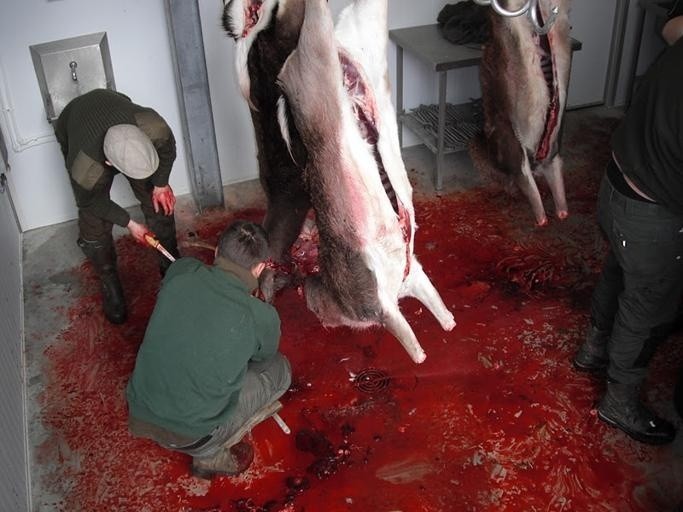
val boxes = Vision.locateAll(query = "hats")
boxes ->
[103,124,159,180]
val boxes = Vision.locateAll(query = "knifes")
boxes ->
[145,234,176,264]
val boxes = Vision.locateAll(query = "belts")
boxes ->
[165,426,219,451]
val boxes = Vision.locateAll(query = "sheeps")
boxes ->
[221,0,311,304]
[478,0,571,228]
[276,0,457,365]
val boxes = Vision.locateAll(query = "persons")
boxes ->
[125,222,293,478]
[571,15,683,444]
[53,88,183,325]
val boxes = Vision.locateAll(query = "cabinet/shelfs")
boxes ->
[388,25,583,193]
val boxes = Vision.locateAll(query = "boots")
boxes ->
[191,400,283,480]
[573,334,610,371]
[597,379,676,445]
[77,237,127,325]
[151,232,181,280]
